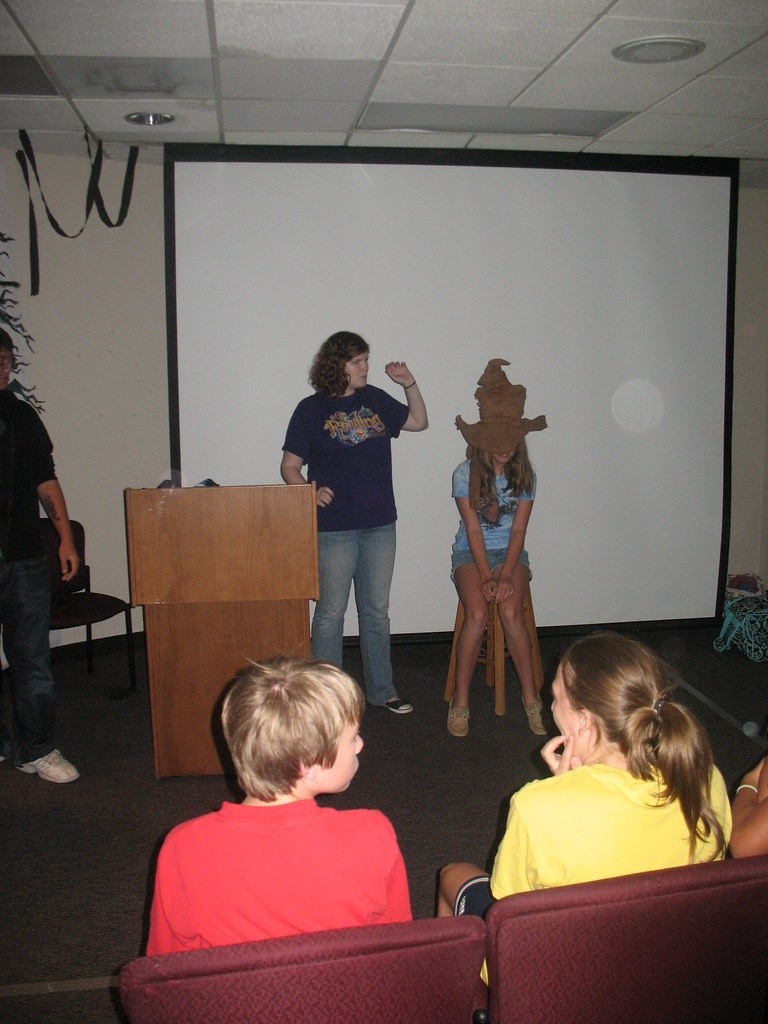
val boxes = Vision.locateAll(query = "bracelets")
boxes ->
[736,785,758,793]
[405,381,415,389]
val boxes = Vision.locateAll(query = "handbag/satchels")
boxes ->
[721,573,768,617]
[713,594,768,663]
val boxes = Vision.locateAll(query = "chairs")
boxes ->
[37,516,139,694]
[120,913,486,1024]
[486,854,768,1024]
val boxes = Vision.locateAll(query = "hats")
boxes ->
[453,359,548,455]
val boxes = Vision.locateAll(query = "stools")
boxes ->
[444,583,543,717]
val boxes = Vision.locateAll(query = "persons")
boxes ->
[447,412,553,736]
[435,633,733,985]
[144,657,413,953]
[281,330,429,713]
[0,329,79,783]
[729,756,768,859]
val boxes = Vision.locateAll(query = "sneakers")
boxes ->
[14,749,79,783]
[447,696,470,737]
[521,692,553,735]
[383,699,413,713]
